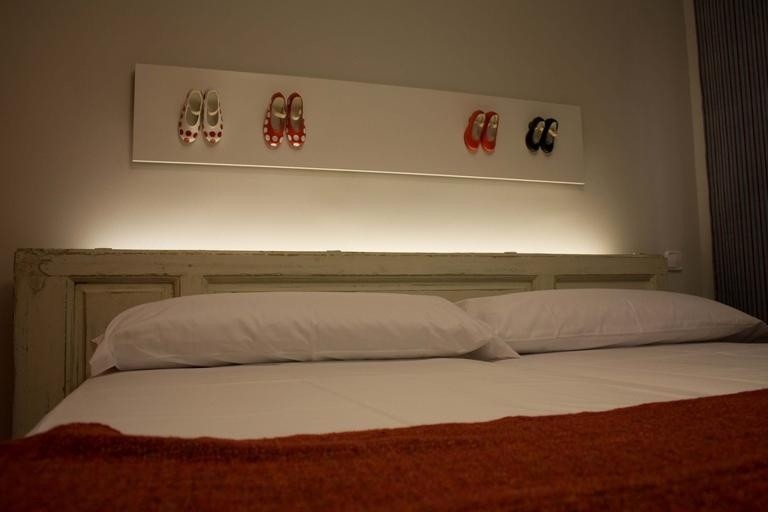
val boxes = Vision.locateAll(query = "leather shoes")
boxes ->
[263,92,287,150]
[541,119,558,153]
[178,88,204,143]
[464,110,486,151]
[203,88,223,144]
[481,112,499,152]
[286,92,306,148]
[525,117,546,152]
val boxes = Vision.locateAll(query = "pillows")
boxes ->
[451,288,768,359]
[86,289,523,380]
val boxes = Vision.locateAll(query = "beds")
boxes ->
[6,244,768,512]
